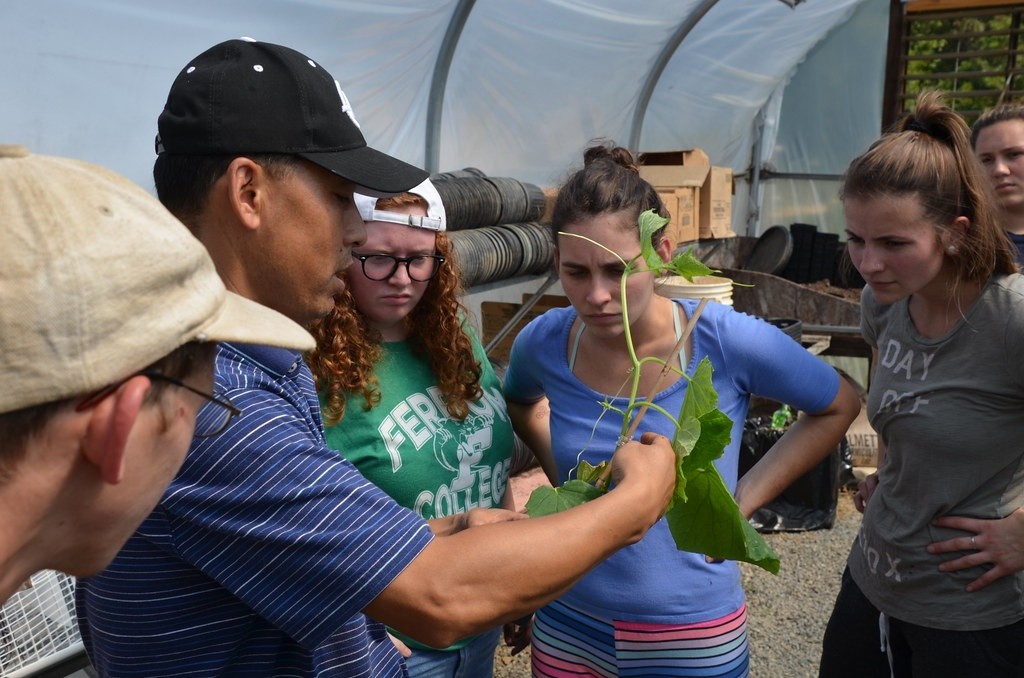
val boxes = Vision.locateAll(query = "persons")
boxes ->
[501,138,860,678]
[77,37,676,678]
[818,87,1024,678]
[0,144,316,609]
[303,176,532,678]
[970,105,1024,274]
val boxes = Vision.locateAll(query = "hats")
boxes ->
[154,37,431,197]
[352,175,447,232]
[0,144,316,415]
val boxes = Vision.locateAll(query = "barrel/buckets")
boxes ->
[653,275,734,308]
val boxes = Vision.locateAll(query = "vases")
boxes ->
[741,317,842,532]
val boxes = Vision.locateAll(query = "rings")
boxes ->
[972,537,977,552]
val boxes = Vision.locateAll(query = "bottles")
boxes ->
[771,403,793,429]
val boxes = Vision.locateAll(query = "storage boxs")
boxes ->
[634,146,737,247]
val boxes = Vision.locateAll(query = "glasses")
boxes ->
[350,250,444,283]
[77,371,241,438]
